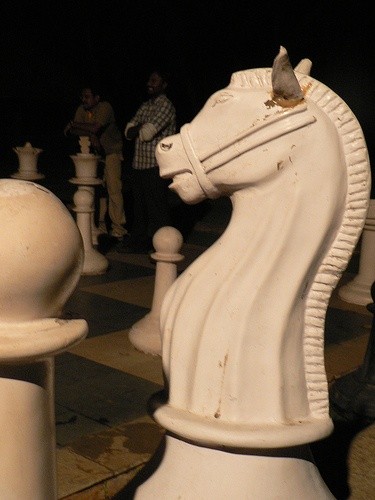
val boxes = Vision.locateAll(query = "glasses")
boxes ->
[80,95,93,99]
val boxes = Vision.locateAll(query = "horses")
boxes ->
[155,45,371,448]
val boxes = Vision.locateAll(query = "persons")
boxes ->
[64,84,129,247]
[116,69,178,253]
